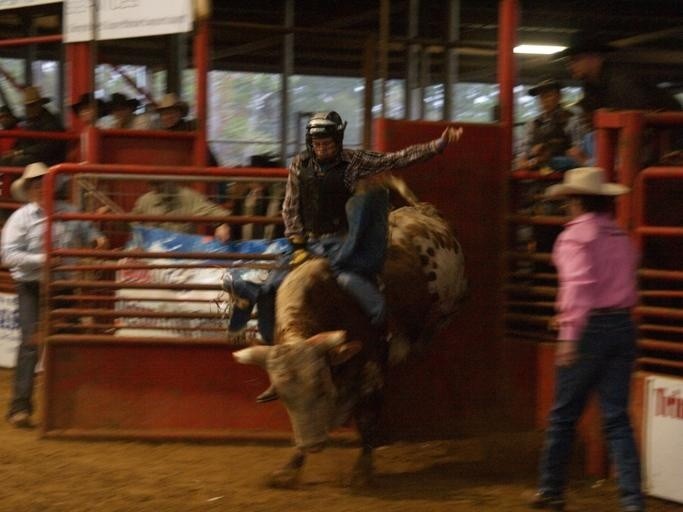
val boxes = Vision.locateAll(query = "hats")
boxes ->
[15,85,51,107]
[71,92,190,117]
[528,77,568,96]
[11,162,61,200]
[544,167,632,200]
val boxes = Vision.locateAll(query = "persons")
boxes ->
[518,74,583,330]
[528,166,644,512]
[230,154,286,340]
[0,82,198,166]
[0,162,111,427]
[135,175,234,245]
[557,32,682,161]
[261,109,465,397]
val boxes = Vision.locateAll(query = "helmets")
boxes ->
[306,110,347,137]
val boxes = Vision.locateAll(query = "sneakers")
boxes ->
[256,384,279,403]
[528,487,564,509]
[385,328,413,368]
[7,408,30,429]
[621,494,645,512]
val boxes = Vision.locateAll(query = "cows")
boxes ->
[229,174,468,497]
